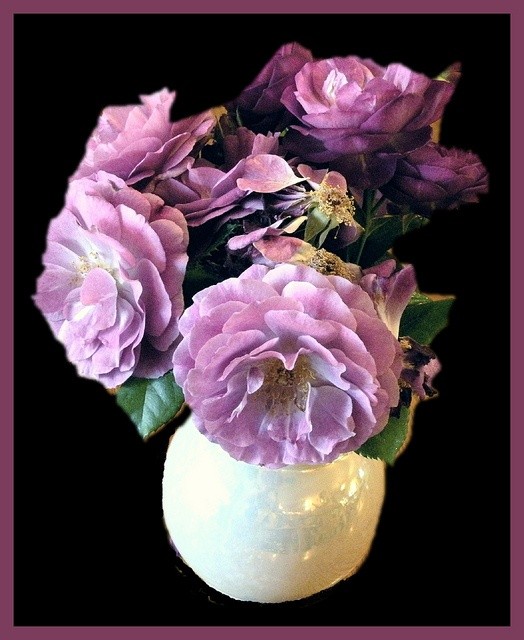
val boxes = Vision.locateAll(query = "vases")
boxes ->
[160,413,386,605]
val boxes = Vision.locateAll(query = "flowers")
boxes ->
[31,44,488,466]
